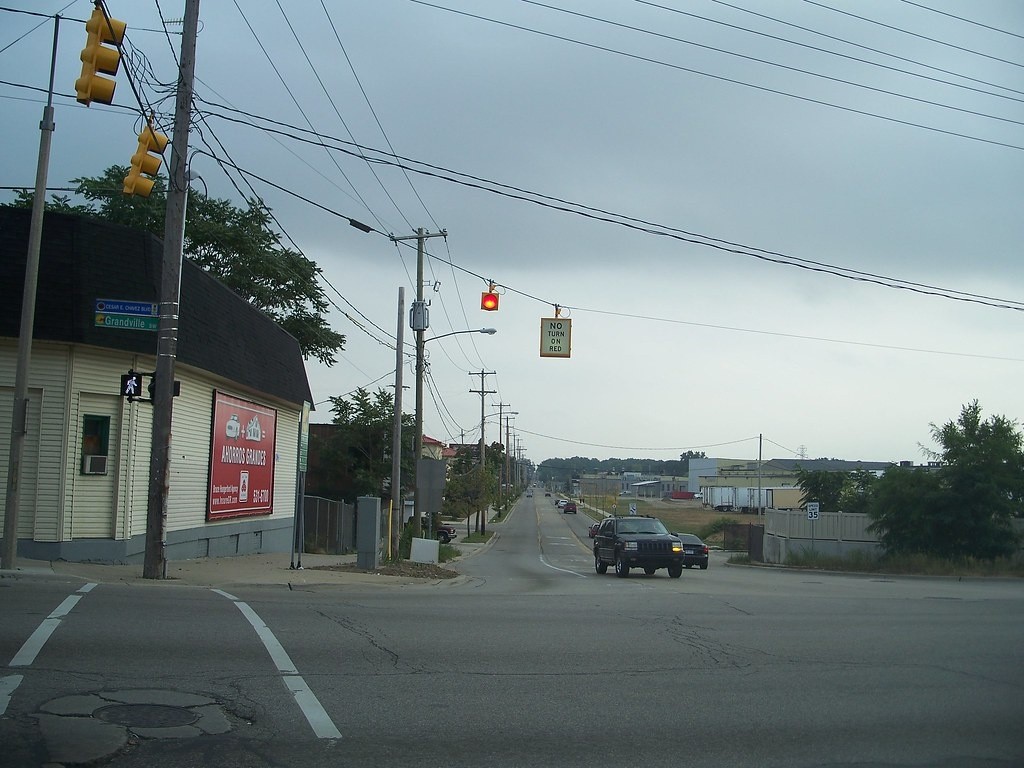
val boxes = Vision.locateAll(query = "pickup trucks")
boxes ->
[405,515,458,544]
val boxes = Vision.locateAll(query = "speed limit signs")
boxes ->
[807,503,820,520]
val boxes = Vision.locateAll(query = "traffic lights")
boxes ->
[483,291,497,311]
[121,376,143,396]
[74,6,125,108]
[121,117,168,197]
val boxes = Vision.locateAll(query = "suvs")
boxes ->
[564,502,577,514]
[594,515,685,578]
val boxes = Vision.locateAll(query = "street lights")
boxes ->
[413,326,497,533]
[481,411,519,534]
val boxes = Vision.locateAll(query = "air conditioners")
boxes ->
[83,454,108,475]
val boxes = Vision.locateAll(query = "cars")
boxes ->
[557,500,568,508]
[588,524,602,537]
[693,491,703,499]
[554,500,561,505]
[671,531,710,570]
[527,484,552,499]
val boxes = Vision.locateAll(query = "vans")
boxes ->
[618,491,631,496]
